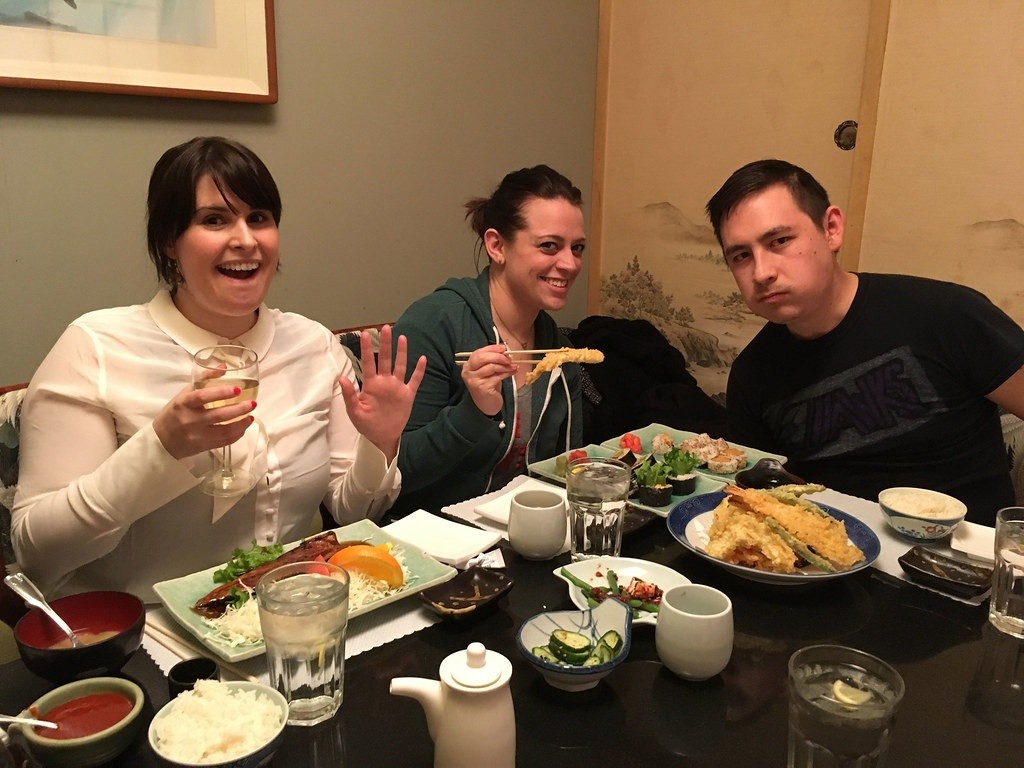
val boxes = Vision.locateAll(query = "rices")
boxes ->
[153,677,283,764]
[881,490,960,519]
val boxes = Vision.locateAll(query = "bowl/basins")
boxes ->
[147,680,290,768]
[878,487,968,545]
[515,596,633,693]
[13,591,148,684]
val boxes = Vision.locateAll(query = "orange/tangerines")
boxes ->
[326,545,403,589]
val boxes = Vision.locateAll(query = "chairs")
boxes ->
[0,322,398,665]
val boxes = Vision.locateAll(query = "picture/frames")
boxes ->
[0,0,279,106]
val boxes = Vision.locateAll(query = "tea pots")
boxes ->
[389,642,516,768]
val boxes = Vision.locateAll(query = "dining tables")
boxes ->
[0,480,1024,768]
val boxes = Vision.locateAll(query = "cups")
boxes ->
[988,506,1024,640]
[787,645,905,768]
[653,583,733,679]
[508,490,567,562]
[3,677,155,768]
[566,457,631,563]
[169,657,219,700]
[256,561,350,727]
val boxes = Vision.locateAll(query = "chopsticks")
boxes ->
[454,349,567,365]
[143,619,255,680]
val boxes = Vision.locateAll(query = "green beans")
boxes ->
[561,567,660,618]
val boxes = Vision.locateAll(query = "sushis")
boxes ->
[651,433,747,475]
[609,448,698,506]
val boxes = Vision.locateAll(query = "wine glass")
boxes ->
[195,345,255,498]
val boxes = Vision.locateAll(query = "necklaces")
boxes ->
[490,298,532,350]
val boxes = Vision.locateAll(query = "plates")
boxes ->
[473,423,880,585]
[898,521,995,600]
[379,509,502,565]
[419,565,517,614]
[552,556,692,626]
[153,519,458,662]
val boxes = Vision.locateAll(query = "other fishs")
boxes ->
[711,484,867,575]
[525,347,605,387]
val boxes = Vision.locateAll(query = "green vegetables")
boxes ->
[214,538,285,608]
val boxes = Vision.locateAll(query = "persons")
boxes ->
[393,164,587,509]
[706,158,1024,525]
[11,136,428,605]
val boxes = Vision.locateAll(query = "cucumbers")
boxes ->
[533,628,624,667]
